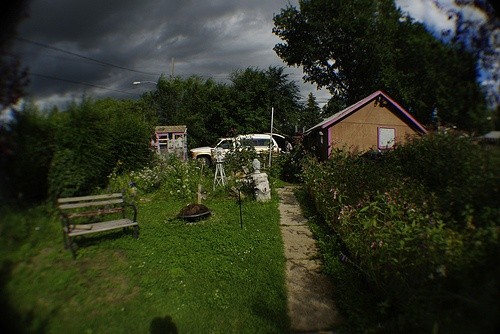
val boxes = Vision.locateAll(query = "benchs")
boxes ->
[57,193,140,259]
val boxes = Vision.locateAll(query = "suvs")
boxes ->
[190,134,280,167]
